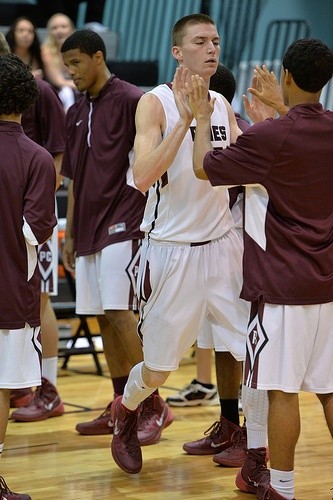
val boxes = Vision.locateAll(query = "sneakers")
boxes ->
[214,427,269,466]
[9,388,32,408]
[167,384,220,407]
[0,476,31,500]
[12,377,64,422]
[136,395,174,446]
[182,416,242,455]
[235,448,270,494]
[76,386,125,435]
[257,482,297,500]
[112,396,143,474]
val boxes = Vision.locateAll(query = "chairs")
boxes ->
[47,252,103,376]
